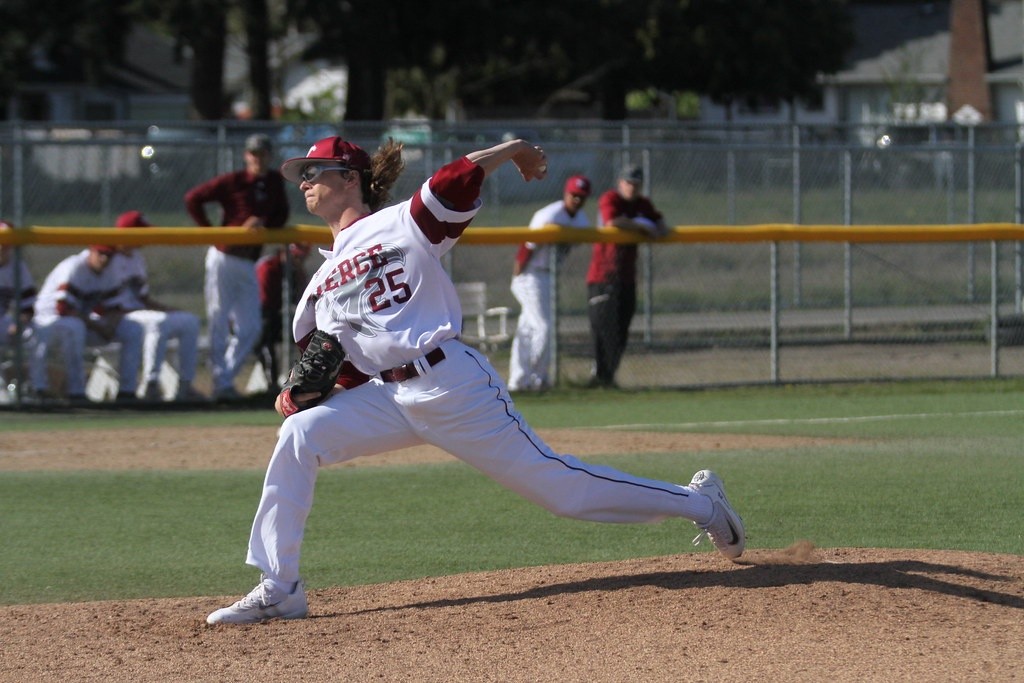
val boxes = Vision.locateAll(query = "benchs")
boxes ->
[85,332,235,398]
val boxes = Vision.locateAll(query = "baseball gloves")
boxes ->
[279,329,345,419]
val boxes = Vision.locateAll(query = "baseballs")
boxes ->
[538,165,546,173]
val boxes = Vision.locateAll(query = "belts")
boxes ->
[380,346,445,382]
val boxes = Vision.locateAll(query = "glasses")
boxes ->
[302,166,353,183]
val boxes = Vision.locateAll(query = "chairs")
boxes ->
[455,284,510,356]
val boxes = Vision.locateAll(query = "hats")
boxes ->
[246,133,272,155]
[564,176,590,197]
[281,135,370,184]
[617,165,642,186]
[94,244,115,254]
[116,212,151,228]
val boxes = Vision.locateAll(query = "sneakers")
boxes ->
[689,469,745,559]
[207,573,309,624]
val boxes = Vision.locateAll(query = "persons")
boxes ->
[508,174,593,393]
[585,165,663,390]
[113,213,206,405]
[0,221,38,353]
[204,133,746,621]
[187,133,291,402]
[253,239,314,392]
[30,242,147,411]
